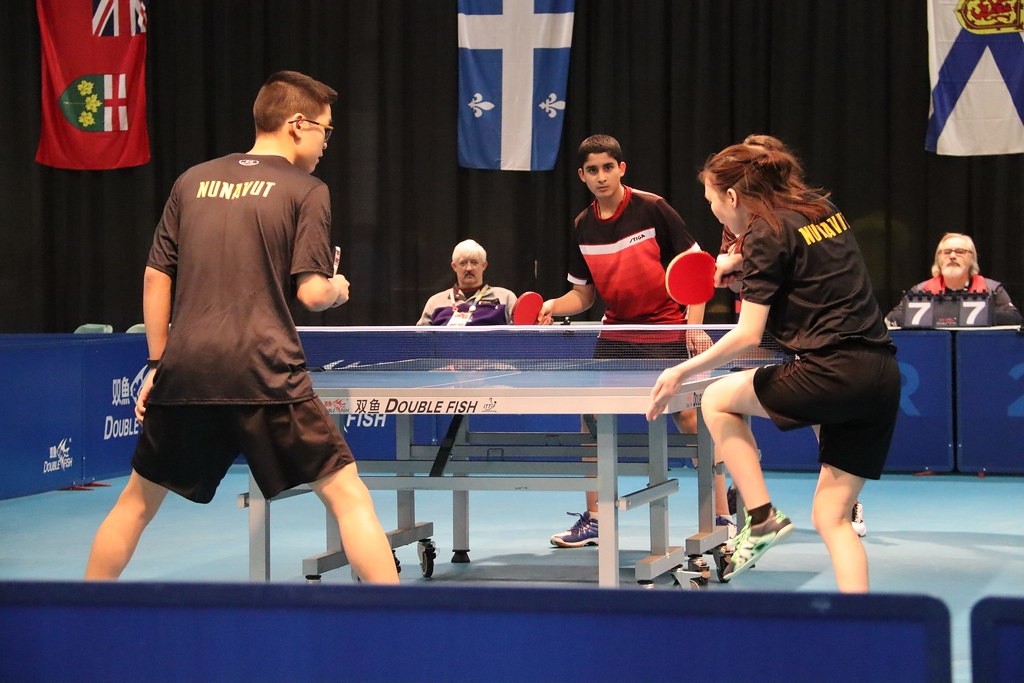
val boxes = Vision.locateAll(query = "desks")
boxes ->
[237,359,752,589]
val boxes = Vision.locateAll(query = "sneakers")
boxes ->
[847,501,866,537]
[720,506,793,582]
[548,511,599,549]
[726,483,738,516]
[715,515,740,543]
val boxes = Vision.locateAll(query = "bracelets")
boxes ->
[147,360,158,369]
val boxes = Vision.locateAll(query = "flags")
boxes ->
[924,0,1024,156]
[34,0,151,170]
[457,0,576,171]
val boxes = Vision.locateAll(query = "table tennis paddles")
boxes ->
[331,245,342,277]
[664,249,742,306]
[511,290,554,326]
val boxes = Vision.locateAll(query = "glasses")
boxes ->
[936,248,974,257]
[287,119,335,142]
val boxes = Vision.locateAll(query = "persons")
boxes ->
[716,134,868,536]
[535,134,738,553]
[645,145,901,594]
[85,73,402,584]
[415,239,518,371]
[884,232,1021,325]
